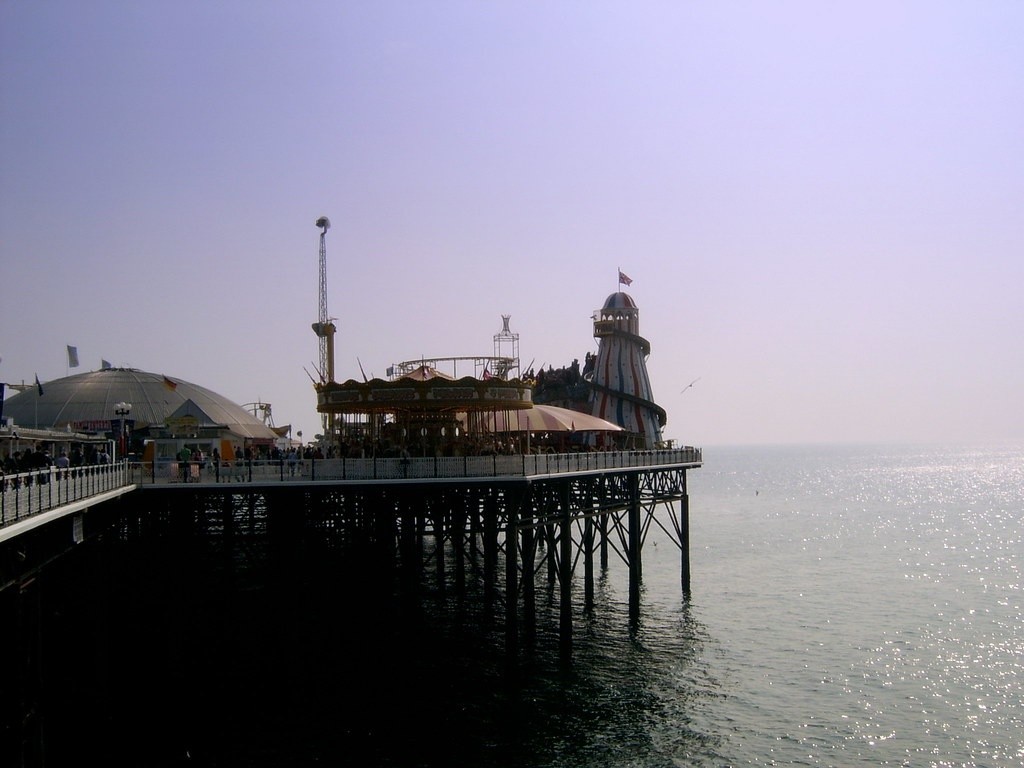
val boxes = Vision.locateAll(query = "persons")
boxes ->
[0,445,111,491]
[176,446,324,483]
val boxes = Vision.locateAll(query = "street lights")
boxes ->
[112,401,133,459]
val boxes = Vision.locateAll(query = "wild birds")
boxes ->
[681,377,701,393]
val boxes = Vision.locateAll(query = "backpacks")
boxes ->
[99,454,107,464]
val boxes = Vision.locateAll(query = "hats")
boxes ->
[100,449,105,453]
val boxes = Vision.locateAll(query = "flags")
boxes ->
[163,376,177,393]
[619,272,633,287]
[36,376,44,397]
